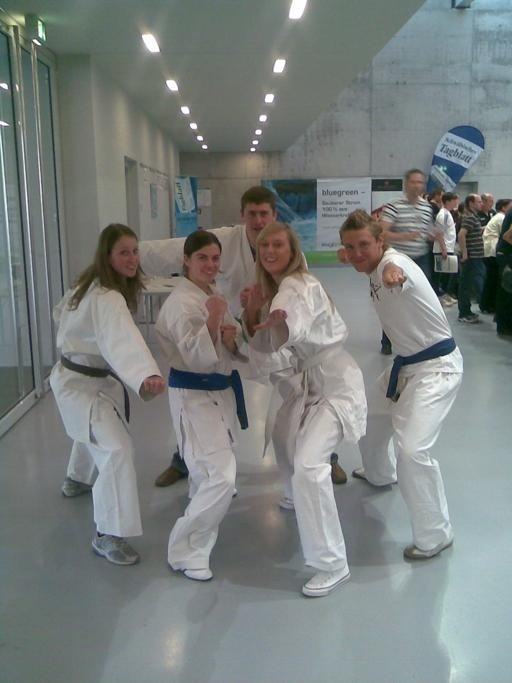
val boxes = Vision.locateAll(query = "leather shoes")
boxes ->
[155,466,182,487]
[381,345,392,354]
[331,454,347,483]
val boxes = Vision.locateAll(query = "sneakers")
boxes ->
[352,467,366,481]
[404,537,453,558]
[62,477,93,496]
[182,568,213,580]
[439,293,458,307]
[279,496,294,509]
[303,564,350,595]
[458,311,479,322]
[92,532,139,564]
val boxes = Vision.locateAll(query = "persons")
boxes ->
[48,223,167,566]
[239,223,367,598]
[139,187,350,486]
[337,208,465,558]
[373,169,510,356]
[156,232,246,583]
